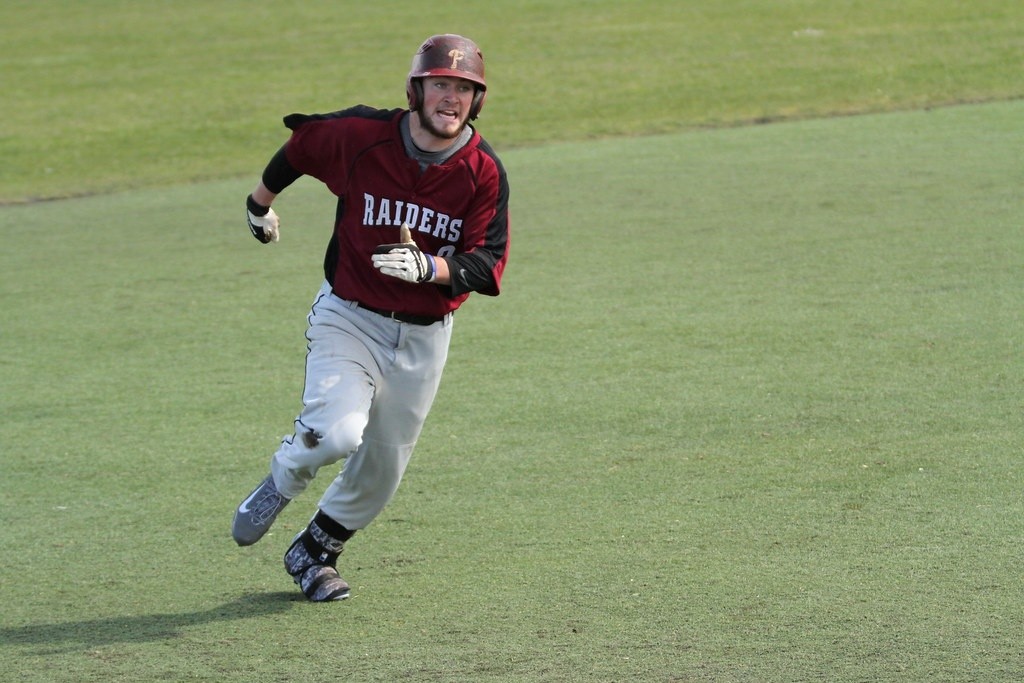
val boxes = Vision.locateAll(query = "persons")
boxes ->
[232,34,510,603]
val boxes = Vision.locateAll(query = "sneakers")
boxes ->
[284,532,352,600]
[232,472,293,546]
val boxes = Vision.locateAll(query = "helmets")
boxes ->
[406,34,488,121]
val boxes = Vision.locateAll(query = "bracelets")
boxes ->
[425,254,436,282]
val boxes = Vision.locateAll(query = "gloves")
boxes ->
[371,221,437,284]
[245,193,280,244]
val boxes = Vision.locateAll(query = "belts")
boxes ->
[331,289,455,326]
